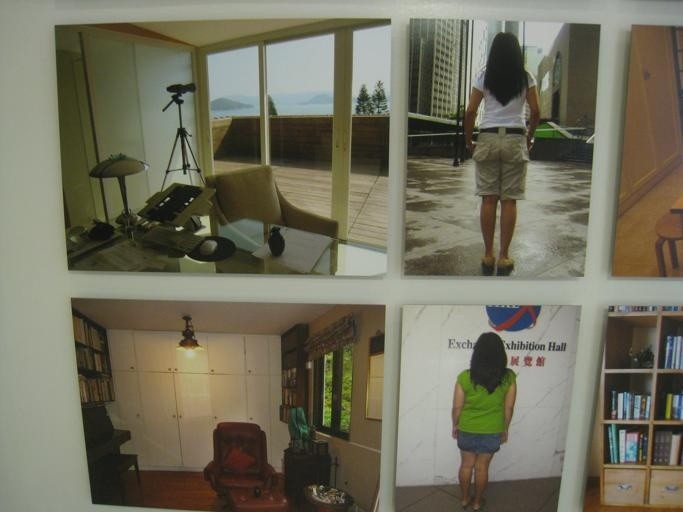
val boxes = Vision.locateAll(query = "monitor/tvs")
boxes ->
[137,183,216,233]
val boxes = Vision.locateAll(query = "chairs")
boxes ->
[204,162,338,276]
[203,422,279,497]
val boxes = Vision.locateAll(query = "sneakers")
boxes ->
[481,252,495,268]
[496,256,515,273]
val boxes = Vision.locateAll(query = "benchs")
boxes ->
[92,454,141,505]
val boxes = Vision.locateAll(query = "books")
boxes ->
[72,313,114,403]
[606,335,683,465]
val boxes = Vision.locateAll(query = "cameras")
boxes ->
[166,83,195,93]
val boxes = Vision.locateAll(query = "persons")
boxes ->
[451,332,518,510]
[464,31,541,271]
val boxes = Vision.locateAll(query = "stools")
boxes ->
[655,214,683,278]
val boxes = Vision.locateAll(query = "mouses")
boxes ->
[199,239,218,256]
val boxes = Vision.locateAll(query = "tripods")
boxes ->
[160,99,206,192]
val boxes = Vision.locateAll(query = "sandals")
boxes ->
[470,496,487,511]
[457,495,472,507]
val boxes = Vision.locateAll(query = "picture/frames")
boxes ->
[363,352,385,422]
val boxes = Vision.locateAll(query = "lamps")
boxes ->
[176,316,206,358]
[90,154,150,226]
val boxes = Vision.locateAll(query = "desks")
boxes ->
[283,446,331,501]
[66,212,388,279]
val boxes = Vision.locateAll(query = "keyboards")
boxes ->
[141,226,206,254]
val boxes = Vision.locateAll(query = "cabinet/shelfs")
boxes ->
[72,306,115,407]
[278,323,309,423]
[597,305,683,511]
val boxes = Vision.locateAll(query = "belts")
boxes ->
[478,127,527,133]
